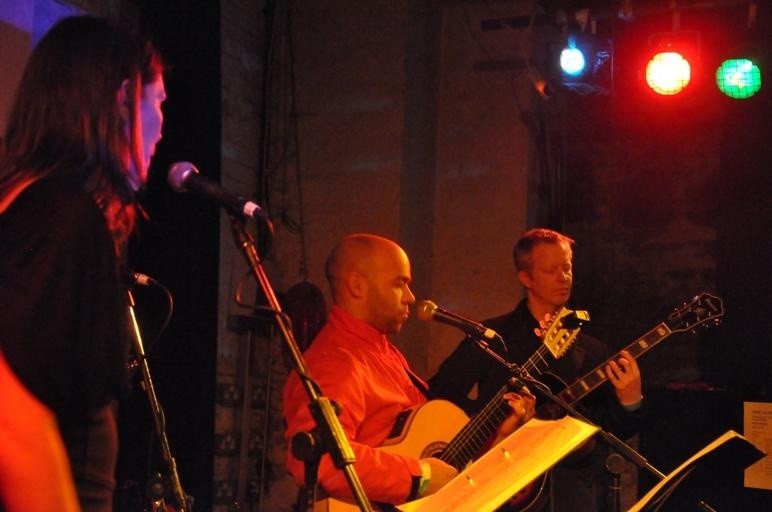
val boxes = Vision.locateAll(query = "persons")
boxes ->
[422,228,651,512]
[1,15,167,512]
[281,234,525,512]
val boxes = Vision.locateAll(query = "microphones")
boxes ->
[418,300,496,344]
[168,161,262,222]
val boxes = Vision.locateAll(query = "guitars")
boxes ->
[472,292,724,512]
[313,303,582,511]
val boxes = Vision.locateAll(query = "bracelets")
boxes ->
[419,461,431,497]
